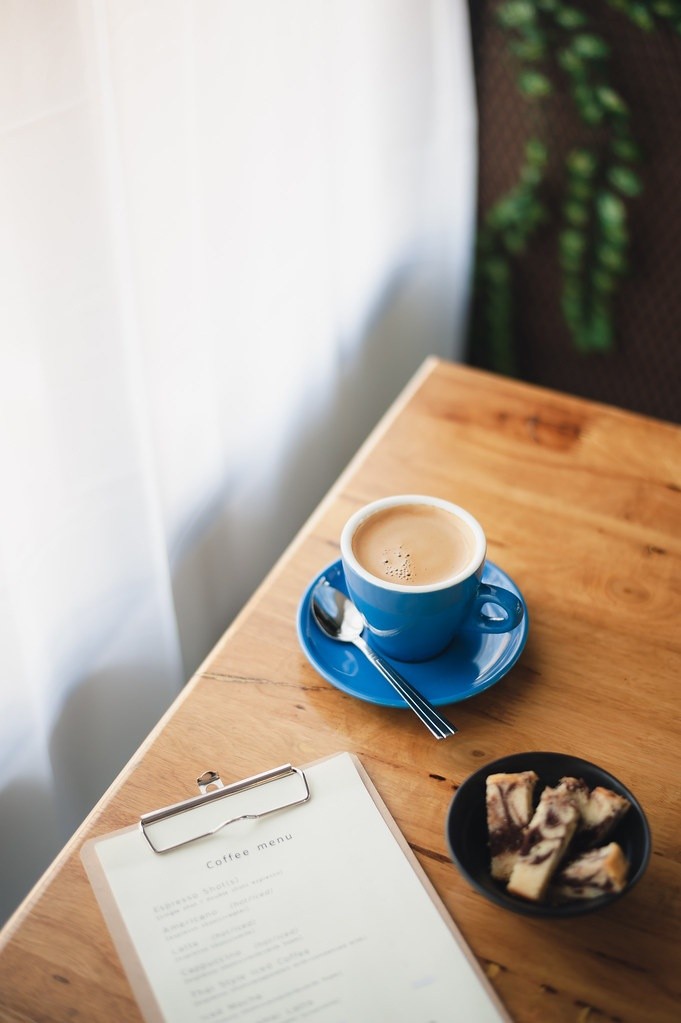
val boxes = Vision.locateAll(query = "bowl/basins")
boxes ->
[444,751,651,919]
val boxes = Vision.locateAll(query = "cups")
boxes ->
[340,495,524,665]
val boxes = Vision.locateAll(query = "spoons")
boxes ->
[312,585,459,740]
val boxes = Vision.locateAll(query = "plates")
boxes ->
[297,557,528,708]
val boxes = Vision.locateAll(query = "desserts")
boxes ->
[484,773,632,903]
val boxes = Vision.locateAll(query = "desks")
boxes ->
[0,354,681,1023]
